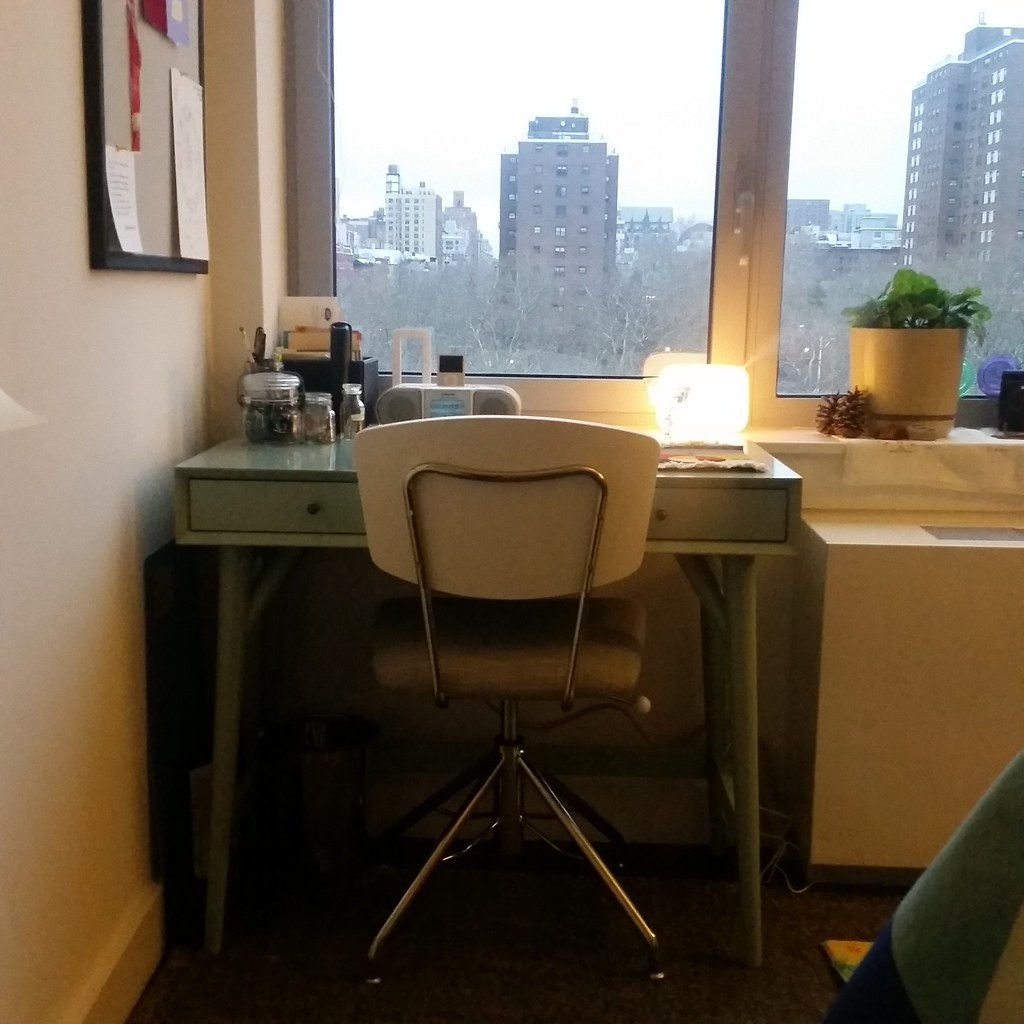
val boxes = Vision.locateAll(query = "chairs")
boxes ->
[339,413,665,987]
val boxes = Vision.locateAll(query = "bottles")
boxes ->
[339,383,365,439]
[241,372,301,445]
[302,392,337,443]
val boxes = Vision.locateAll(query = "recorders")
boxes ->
[378,383,523,425]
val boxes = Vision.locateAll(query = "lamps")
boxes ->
[653,358,759,446]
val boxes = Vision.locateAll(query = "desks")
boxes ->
[177,434,806,978]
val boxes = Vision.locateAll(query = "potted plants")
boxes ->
[844,268,993,443]
